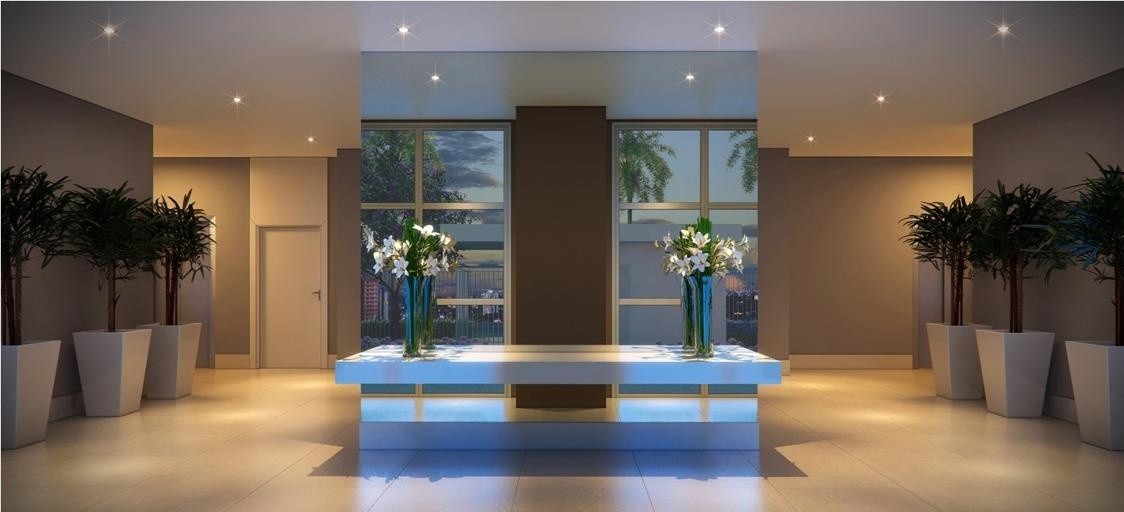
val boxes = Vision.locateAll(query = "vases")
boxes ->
[400,273,435,359]
[679,276,713,359]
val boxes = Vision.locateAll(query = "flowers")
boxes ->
[359,215,464,360]
[654,217,751,358]
